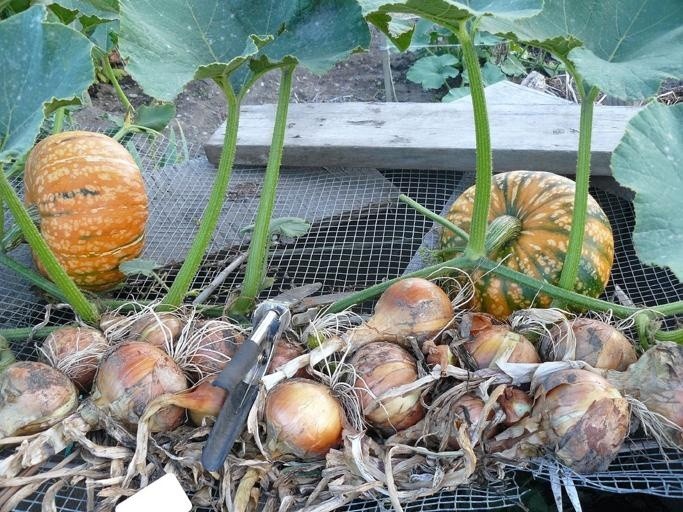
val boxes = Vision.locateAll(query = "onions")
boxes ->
[0,278,683,474]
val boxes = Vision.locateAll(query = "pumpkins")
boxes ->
[440,170,614,342]
[0,131,149,292]
